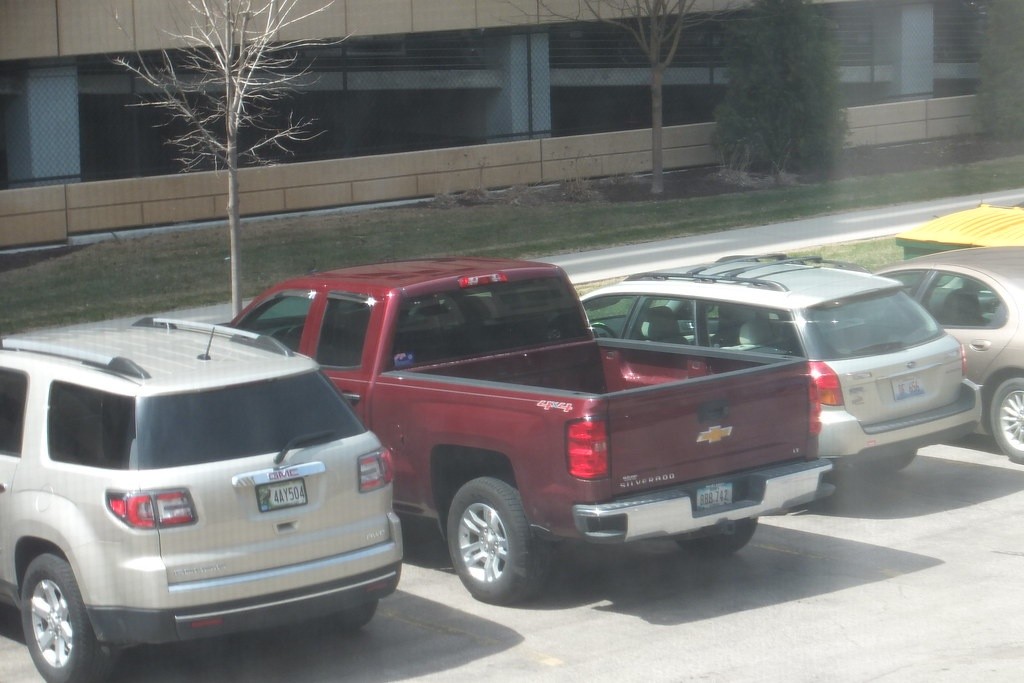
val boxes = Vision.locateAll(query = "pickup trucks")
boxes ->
[218,256,837,607]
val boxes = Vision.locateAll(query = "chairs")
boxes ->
[935,289,990,325]
[725,320,783,351]
[639,305,688,343]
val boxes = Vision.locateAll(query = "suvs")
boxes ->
[583,257,982,481]
[0,316,402,683]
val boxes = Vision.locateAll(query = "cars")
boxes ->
[870,247,1024,463]
